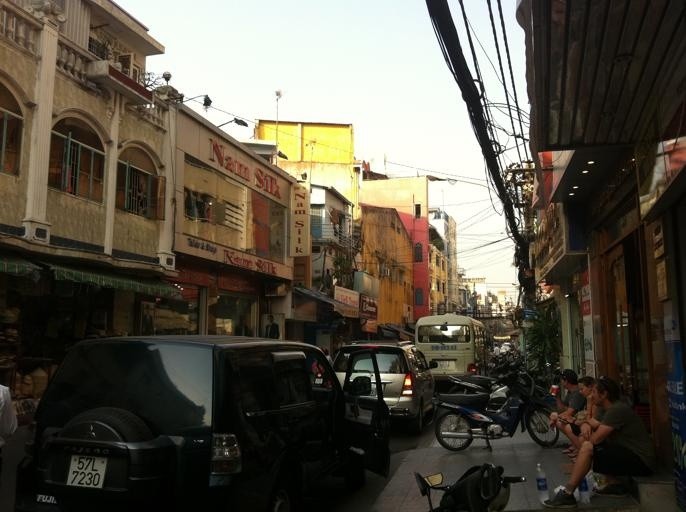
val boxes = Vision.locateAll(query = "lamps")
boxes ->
[217,117,248,130]
[161,71,171,85]
[182,93,211,109]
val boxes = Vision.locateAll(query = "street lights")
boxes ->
[424,173,538,330]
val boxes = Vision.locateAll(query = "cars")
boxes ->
[500,342,513,353]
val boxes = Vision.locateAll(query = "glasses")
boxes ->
[599,376,606,382]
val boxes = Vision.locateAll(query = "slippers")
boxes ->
[561,448,576,453]
[568,452,579,458]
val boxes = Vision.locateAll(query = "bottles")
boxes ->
[535,462,549,501]
[578,470,592,505]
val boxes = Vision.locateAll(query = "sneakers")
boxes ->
[592,483,629,497]
[544,489,577,508]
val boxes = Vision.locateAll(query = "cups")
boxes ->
[551,385,561,396]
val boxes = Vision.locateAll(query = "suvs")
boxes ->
[325,339,440,435]
[13,334,390,510]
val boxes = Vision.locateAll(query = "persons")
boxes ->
[492,343,501,357]
[541,368,654,511]
[0,382,19,493]
[262,316,279,339]
[141,304,154,334]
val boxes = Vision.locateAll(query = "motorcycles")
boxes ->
[414,461,527,511]
[312,372,326,388]
[431,348,560,450]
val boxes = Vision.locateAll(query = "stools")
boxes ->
[628,476,678,512]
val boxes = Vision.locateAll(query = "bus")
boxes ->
[414,311,494,380]
